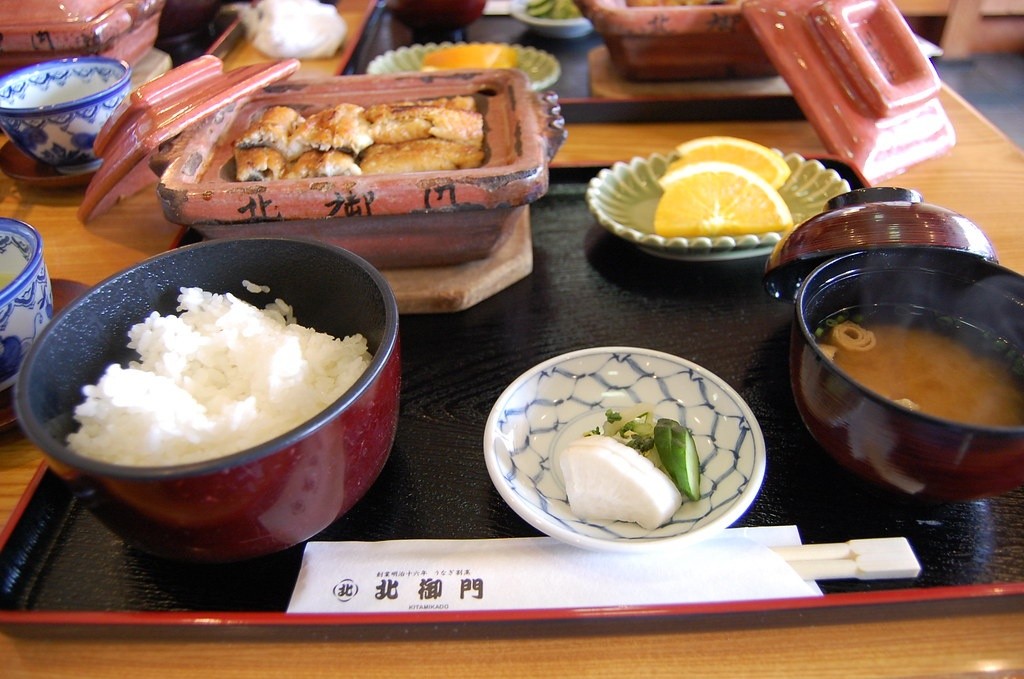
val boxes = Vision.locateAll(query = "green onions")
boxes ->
[813,309,1024,378]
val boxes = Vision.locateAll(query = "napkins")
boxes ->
[232,0,344,61]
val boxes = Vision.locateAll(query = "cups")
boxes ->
[0,218,54,409]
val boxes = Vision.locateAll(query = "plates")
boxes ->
[367,41,562,93]
[125,45,174,95]
[482,346,768,553]
[203,0,381,76]
[0,138,98,189]
[0,280,92,429]
[585,150,849,264]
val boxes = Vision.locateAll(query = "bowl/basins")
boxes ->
[386,0,489,43]
[509,0,595,39]
[149,69,567,267]
[143,0,226,46]
[13,235,403,567]
[764,186,999,303]
[0,56,131,175]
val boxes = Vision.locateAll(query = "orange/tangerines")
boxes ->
[419,43,518,70]
[651,135,792,238]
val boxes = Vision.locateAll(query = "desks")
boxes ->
[0,0,1024,679]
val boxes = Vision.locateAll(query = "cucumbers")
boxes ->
[653,419,706,504]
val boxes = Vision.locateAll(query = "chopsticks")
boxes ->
[773,536,921,580]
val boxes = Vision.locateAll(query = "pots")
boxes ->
[0,0,165,74]
[786,245,1024,506]
[573,0,875,82]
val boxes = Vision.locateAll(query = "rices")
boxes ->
[66,279,375,467]
